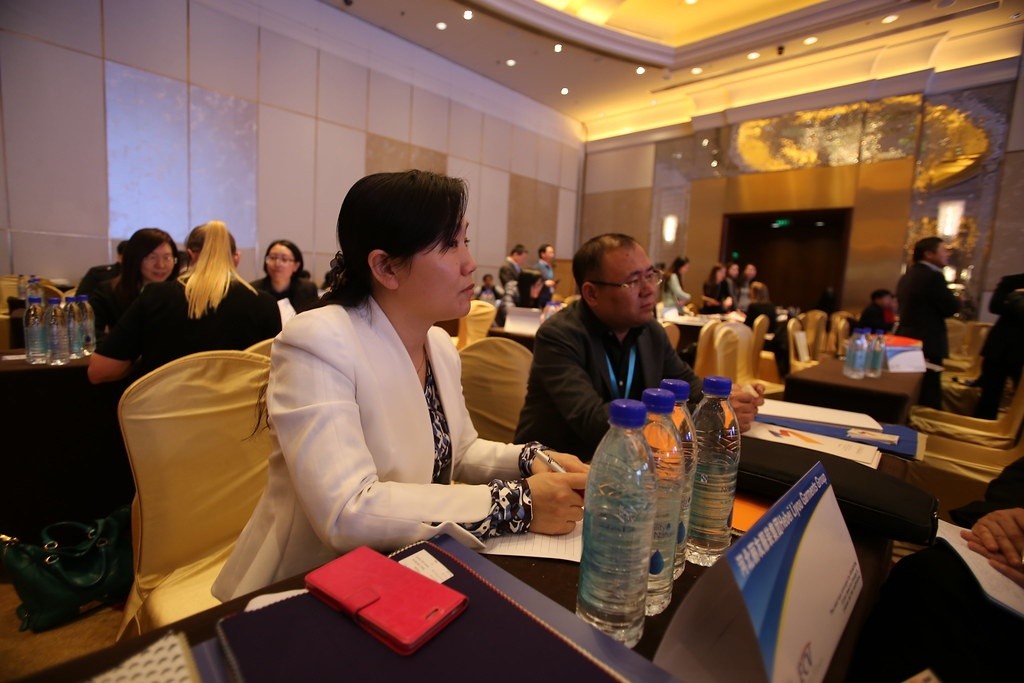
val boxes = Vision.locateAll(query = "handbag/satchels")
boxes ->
[0,520,136,632]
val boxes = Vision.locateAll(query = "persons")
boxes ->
[844,454,1024,683]
[478,241,557,326]
[817,285,835,316]
[210,169,595,606]
[300,270,311,279]
[76,222,280,517]
[248,239,318,311]
[652,253,776,334]
[858,288,899,332]
[979,273,1024,421]
[514,233,764,461]
[893,237,971,409]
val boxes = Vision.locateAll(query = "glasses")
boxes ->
[266,251,297,266]
[587,269,665,292]
[143,253,180,266]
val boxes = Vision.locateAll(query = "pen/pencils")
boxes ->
[531,446,566,473]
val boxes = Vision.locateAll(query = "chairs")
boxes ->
[1,263,1024,683]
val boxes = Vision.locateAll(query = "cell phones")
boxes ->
[305,545,470,656]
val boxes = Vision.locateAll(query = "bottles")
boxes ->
[45,297,68,365]
[16,274,46,364]
[576,399,648,649]
[685,377,741,567]
[63,297,83,361]
[843,328,885,380]
[479,288,495,306]
[643,389,685,616]
[660,379,698,580]
[77,295,96,355]
[540,301,564,321]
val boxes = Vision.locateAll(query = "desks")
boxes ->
[0,342,124,587]
[785,349,937,426]
[13,396,926,683]
